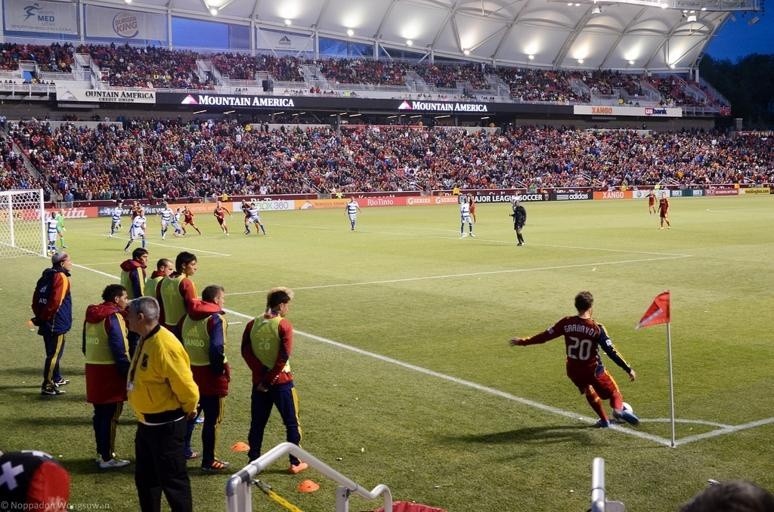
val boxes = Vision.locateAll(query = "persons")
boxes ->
[120,248,148,357]
[157,252,225,423]
[175,284,230,471]
[241,286,309,473]
[0,44,774,256]
[678,480,773,511]
[32,252,73,398]
[125,296,200,511]
[81,284,133,469]
[509,293,639,427]
[144,258,173,299]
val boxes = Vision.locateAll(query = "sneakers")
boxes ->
[188,451,202,458]
[41,388,66,400]
[288,459,309,474]
[596,418,610,427]
[620,409,640,427]
[99,457,131,469]
[201,458,230,474]
[53,378,71,387]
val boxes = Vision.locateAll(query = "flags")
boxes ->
[635,291,670,329]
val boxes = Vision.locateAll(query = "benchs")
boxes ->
[0,32,735,113]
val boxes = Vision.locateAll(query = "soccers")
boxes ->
[613,402,633,423]
